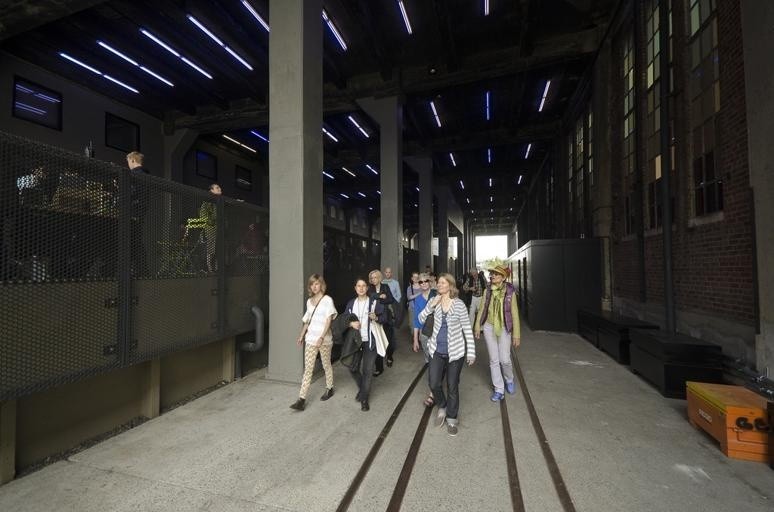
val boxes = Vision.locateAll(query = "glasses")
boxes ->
[418,279,430,284]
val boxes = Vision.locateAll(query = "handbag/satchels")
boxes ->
[422,313,434,338]
[367,298,390,358]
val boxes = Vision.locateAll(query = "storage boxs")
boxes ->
[576,307,774,463]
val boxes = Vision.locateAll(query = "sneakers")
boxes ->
[355,390,362,401]
[387,360,393,368]
[360,400,370,411]
[446,417,459,437]
[491,392,506,402]
[505,382,515,394]
[435,406,447,428]
[319,388,334,401]
[289,397,305,411]
[372,368,383,377]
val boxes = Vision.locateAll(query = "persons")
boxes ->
[475,265,521,402]
[365,269,396,377]
[119,151,149,278]
[16,163,49,194]
[343,278,388,412]
[289,273,338,411]
[418,272,477,437]
[357,242,366,262]
[366,264,488,335]
[324,236,339,266]
[200,183,244,272]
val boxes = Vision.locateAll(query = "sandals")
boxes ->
[424,395,436,408]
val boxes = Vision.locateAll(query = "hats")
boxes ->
[488,265,508,280]
